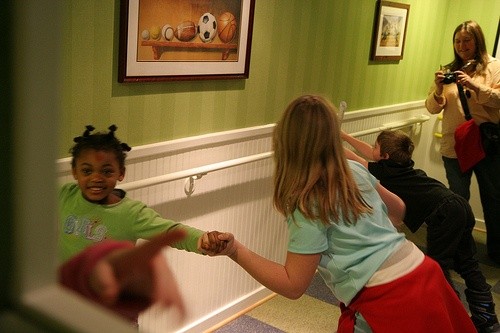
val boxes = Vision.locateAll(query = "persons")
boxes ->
[341,127,499,333]
[425,21,500,268]
[198,94,477,333]
[57,124,226,264]
[58,224,187,325]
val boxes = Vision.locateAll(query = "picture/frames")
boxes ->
[370,0,410,61]
[117,0,257,83]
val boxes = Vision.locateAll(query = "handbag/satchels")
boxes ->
[456,119,485,172]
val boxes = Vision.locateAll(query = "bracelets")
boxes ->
[434,92,442,98]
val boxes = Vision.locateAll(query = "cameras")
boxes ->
[442,73,457,84]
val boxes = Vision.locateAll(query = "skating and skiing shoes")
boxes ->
[464,289,500,333]
